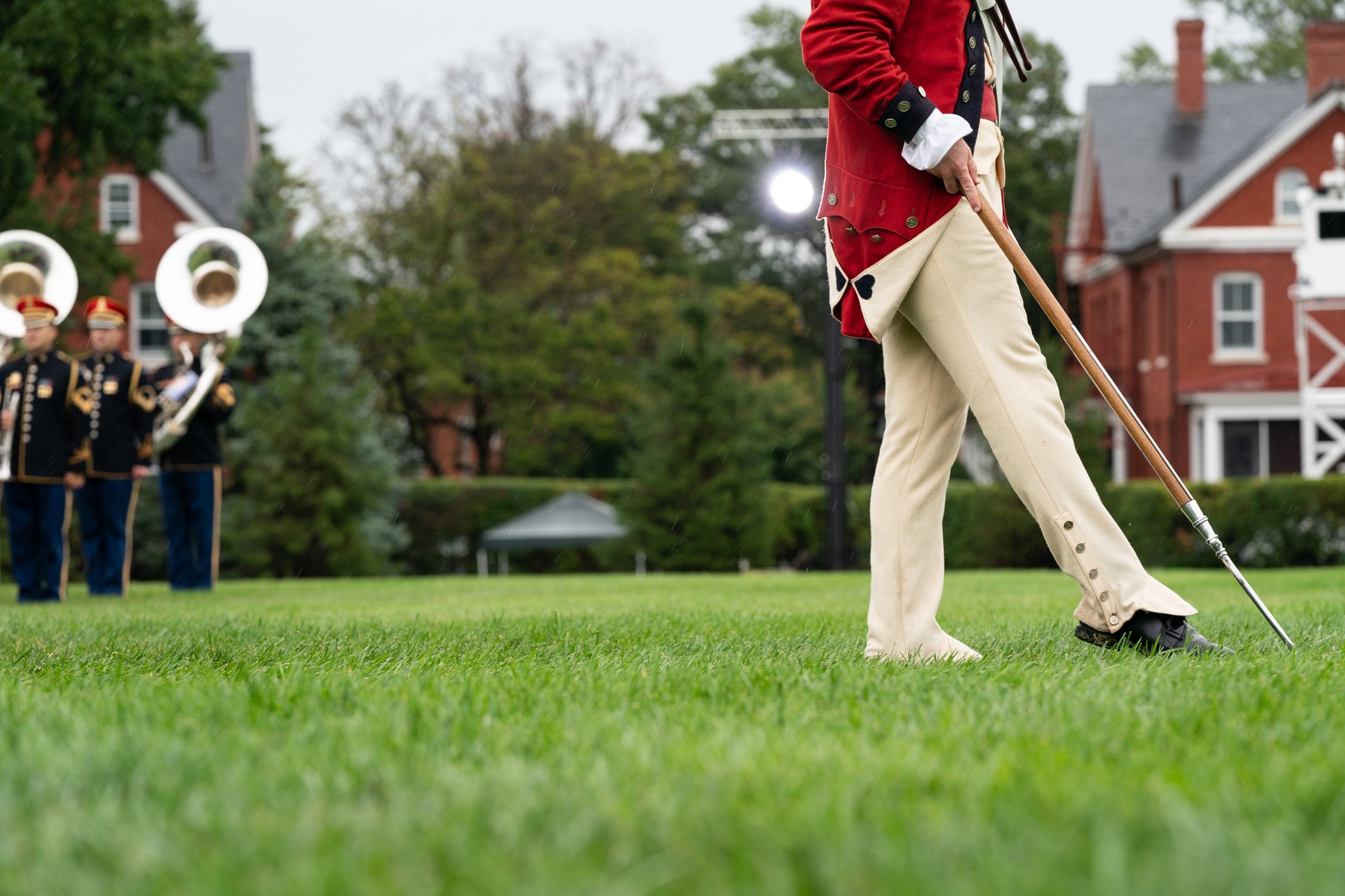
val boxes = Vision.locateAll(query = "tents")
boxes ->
[477,487,646,577]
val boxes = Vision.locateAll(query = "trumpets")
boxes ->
[0,382,21,480]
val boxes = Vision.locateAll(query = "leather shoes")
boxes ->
[1075,609,1235,659]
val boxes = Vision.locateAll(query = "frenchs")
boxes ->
[145,224,270,452]
[0,229,79,371]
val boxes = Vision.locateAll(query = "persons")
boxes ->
[800,0,1243,668]
[0,294,237,606]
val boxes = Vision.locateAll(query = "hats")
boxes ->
[17,300,58,329]
[83,295,130,330]
[164,316,193,335]
